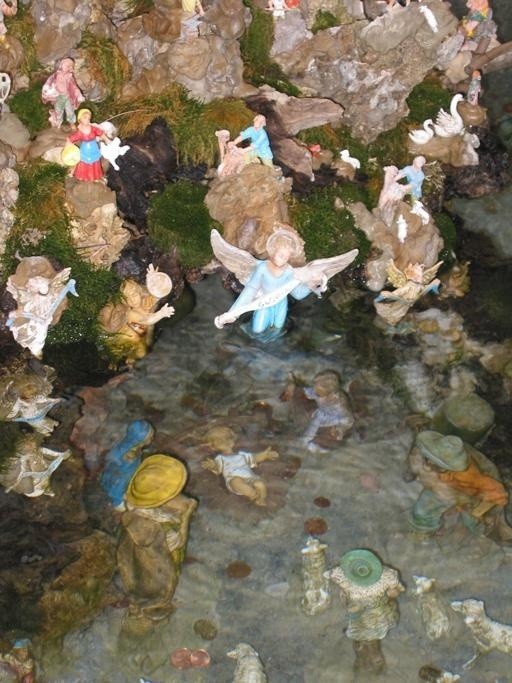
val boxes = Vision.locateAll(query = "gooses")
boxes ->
[433,93,467,138]
[407,117,434,145]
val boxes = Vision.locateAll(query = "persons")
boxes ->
[108,453,198,620]
[403,430,507,546]
[0,0,491,361]
[1,638,38,683]
[322,547,404,644]
[101,371,352,510]
[4,381,66,499]
